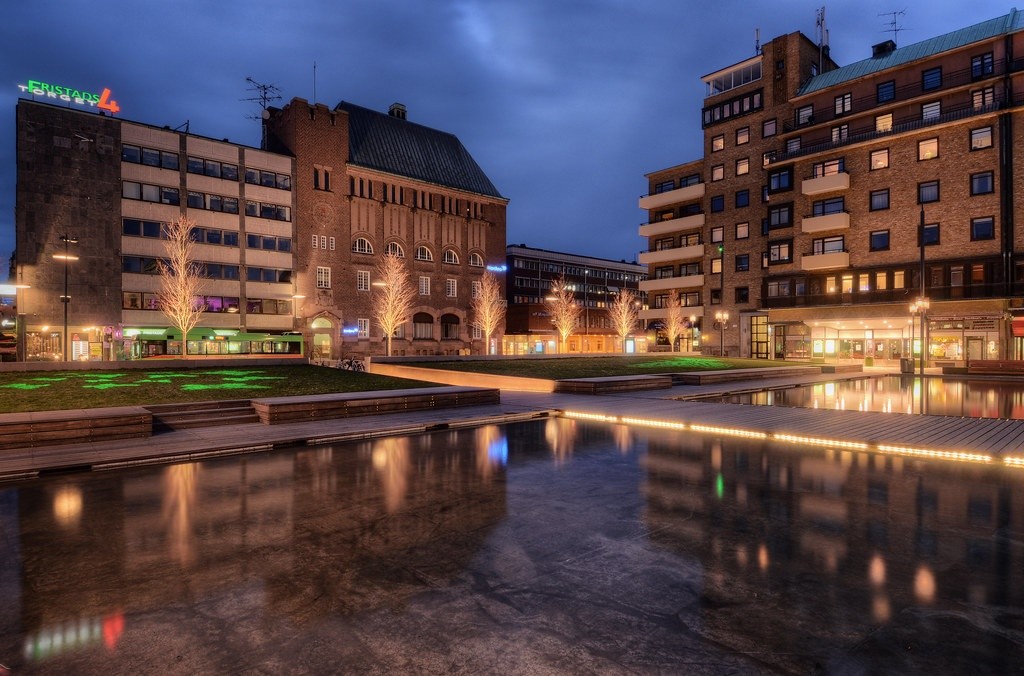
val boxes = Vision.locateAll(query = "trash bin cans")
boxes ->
[900,358,915,374]
[865,357,874,368]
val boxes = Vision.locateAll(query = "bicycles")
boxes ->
[314,354,366,373]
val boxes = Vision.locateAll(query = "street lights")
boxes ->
[909,304,919,359]
[690,314,697,352]
[916,298,930,376]
[715,310,730,358]
[54,229,80,365]
